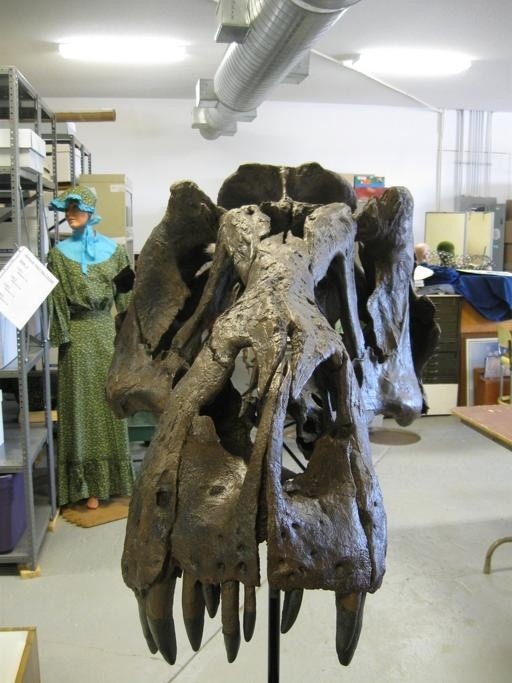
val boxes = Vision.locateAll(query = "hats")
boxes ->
[48,184,96,213]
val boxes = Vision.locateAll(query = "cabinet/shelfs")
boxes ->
[0,64,91,570]
[421,295,466,383]
[425,194,512,276]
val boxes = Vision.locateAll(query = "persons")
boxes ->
[43,185,137,510]
[414,243,431,268]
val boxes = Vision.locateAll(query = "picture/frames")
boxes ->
[459,327,511,406]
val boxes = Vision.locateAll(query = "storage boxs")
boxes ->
[0,471,28,555]
[0,128,89,185]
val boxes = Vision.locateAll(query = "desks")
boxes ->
[450,402,512,575]
